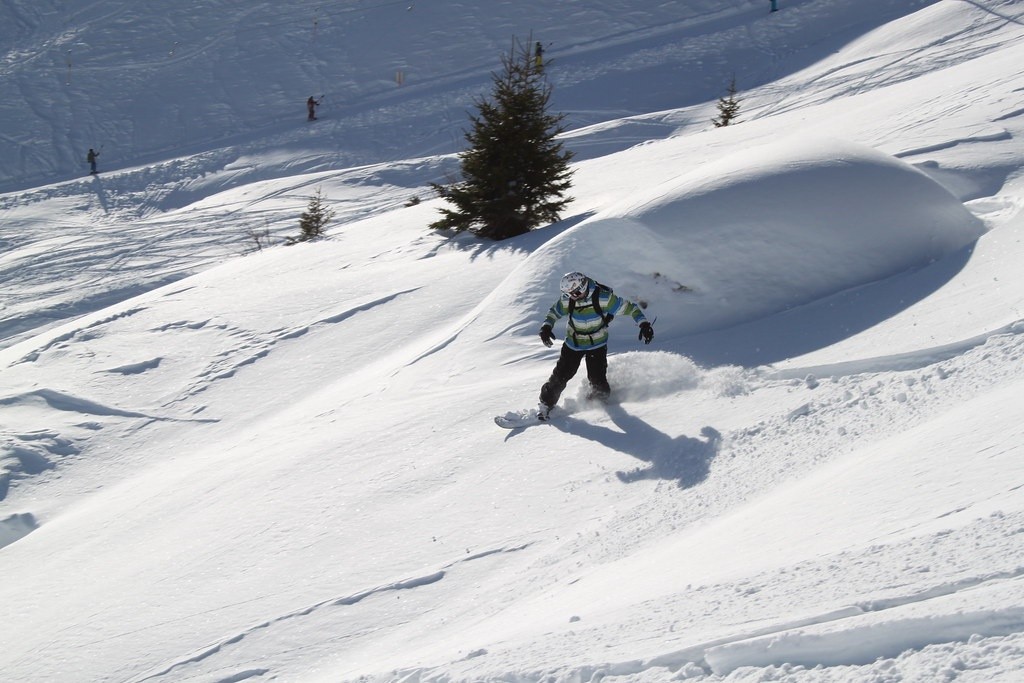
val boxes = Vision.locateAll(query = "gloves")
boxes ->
[639,322,654,344]
[539,325,556,348]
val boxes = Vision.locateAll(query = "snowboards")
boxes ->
[493,405,568,429]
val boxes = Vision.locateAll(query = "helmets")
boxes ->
[560,272,588,300]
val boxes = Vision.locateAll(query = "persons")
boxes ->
[307,95,319,121]
[534,41,545,67]
[770,0,778,12]
[87,148,101,173]
[536,271,654,422]
